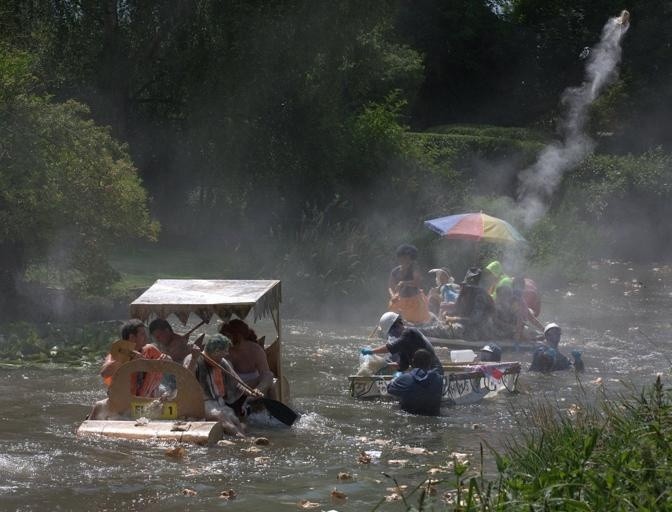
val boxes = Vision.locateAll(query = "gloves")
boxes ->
[361,348,372,355]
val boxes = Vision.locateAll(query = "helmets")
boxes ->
[380,312,400,336]
[543,323,561,337]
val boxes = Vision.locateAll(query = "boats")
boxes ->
[75,279,293,445]
[408,326,547,353]
[344,356,522,406]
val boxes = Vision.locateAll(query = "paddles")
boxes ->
[198,350,299,427]
[111,339,144,364]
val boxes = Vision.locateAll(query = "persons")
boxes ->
[387,244,546,344]
[182,333,265,432]
[100,316,173,397]
[218,318,274,402]
[148,319,189,390]
[528,322,584,372]
[362,311,444,417]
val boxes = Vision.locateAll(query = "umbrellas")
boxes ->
[422,207,528,264]
